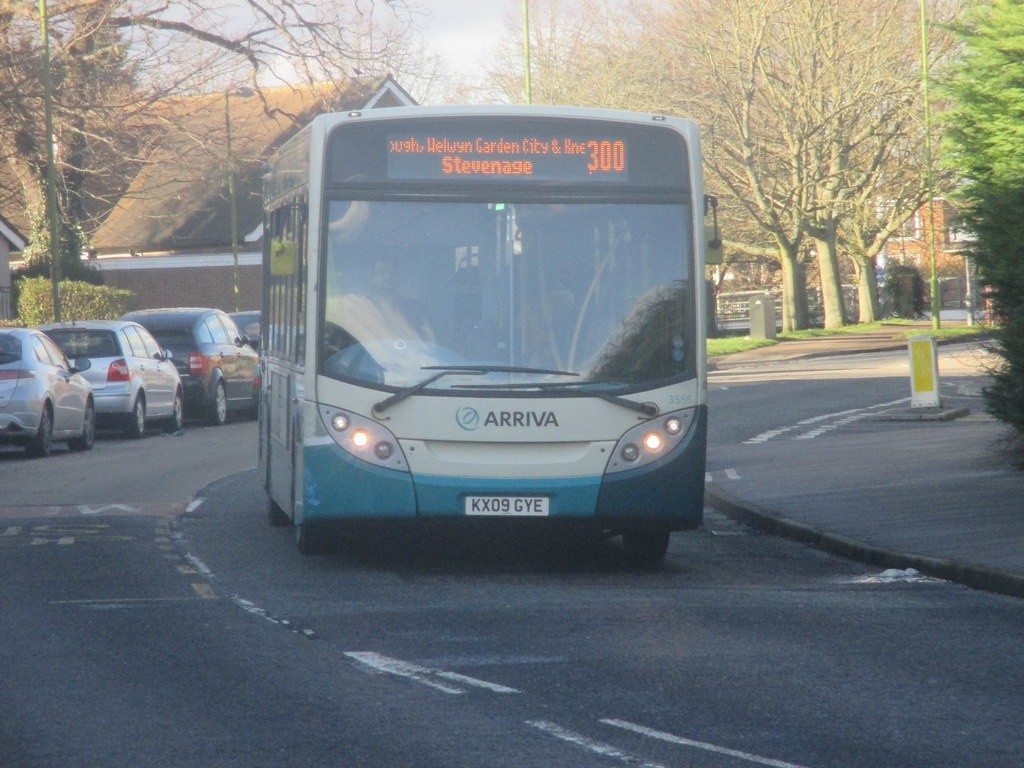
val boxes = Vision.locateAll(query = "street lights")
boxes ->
[222,86,255,313]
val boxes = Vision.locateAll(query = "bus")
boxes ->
[257,103,723,566]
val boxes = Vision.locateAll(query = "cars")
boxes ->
[31,320,185,439]
[0,327,96,458]
[117,307,270,426]
